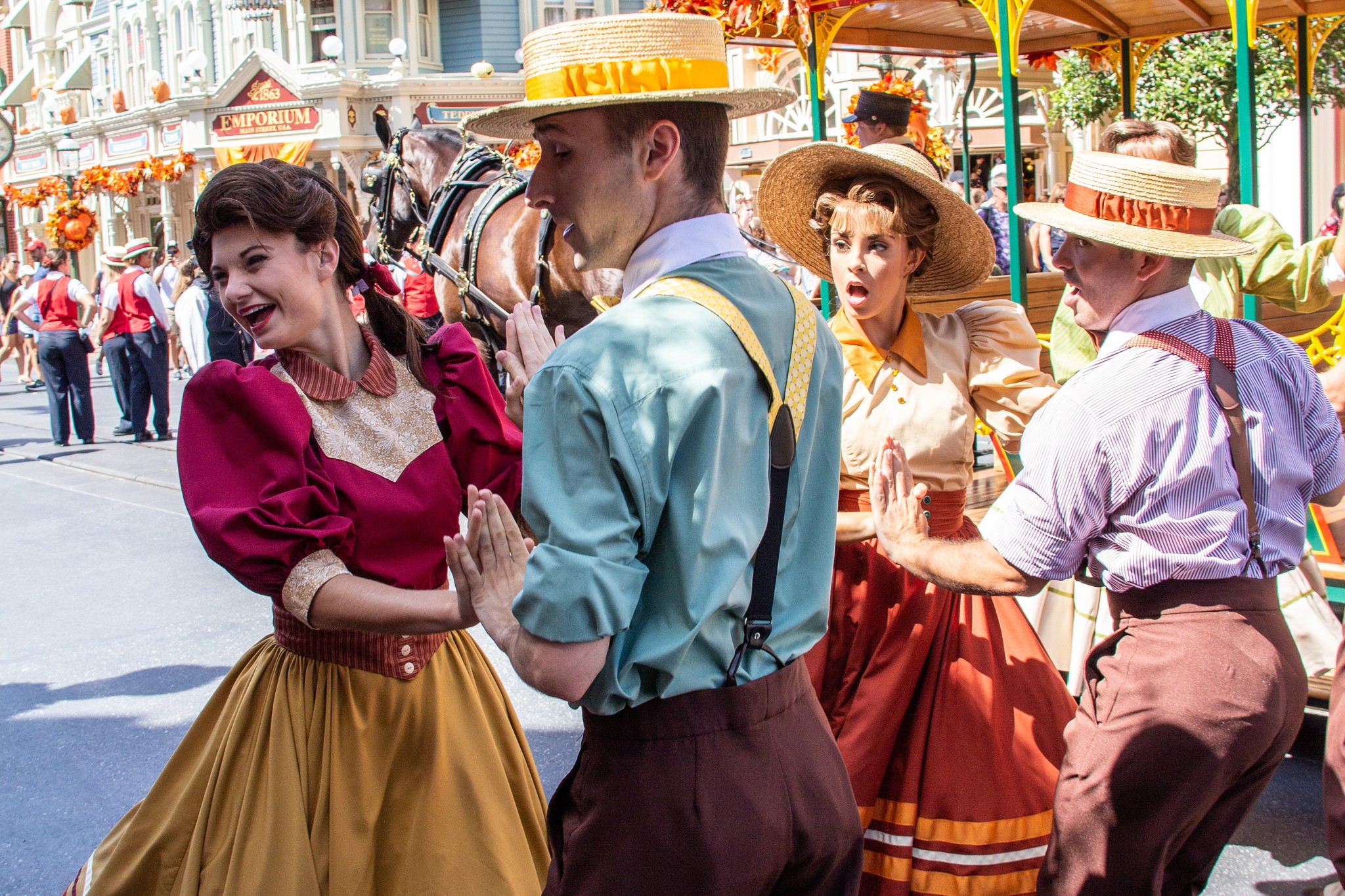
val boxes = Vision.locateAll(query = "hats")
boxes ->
[167,240,177,250]
[18,265,37,278]
[841,90,913,127]
[24,239,46,251]
[1010,148,1256,258]
[754,139,996,298]
[120,237,159,261]
[991,164,1007,178]
[1332,182,1345,206]
[186,239,196,256]
[459,12,800,144]
[405,226,426,250]
[950,170,965,183]
[99,245,131,267]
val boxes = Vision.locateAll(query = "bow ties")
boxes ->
[1083,327,1110,354]
[590,295,622,315]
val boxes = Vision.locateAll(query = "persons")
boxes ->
[723,170,819,298]
[947,162,1011,275]
[98,245,135,436]
[173,241,275,373]
[355,214,445,331]
[0,241,51,391]
[872,150,1345,896]
[1321,619,1345,896]
[1012,118,1345,695]
[843,85,945,185]
[11,248,97,444]
[91,252,107,375]
[153,240,194,379]
[1022,183,1067,272]
[452,17,861,895]
[117,237,172,441]
[1315,182,1345,236]
[757,144,1077,896]
[64,158,548,896]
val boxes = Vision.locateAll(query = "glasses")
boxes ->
[143,251,153,256]
[174,250,178,254]
[68,258,74,264]
[1058,197,1065,203]
[29,249,35,253]
[12,260,20,264]
[995,186,1008,193]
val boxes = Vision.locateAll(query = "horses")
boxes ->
[365,113,624,391]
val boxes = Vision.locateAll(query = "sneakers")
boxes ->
[17,359,196,392]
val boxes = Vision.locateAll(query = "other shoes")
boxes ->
[55,425,172,447]
